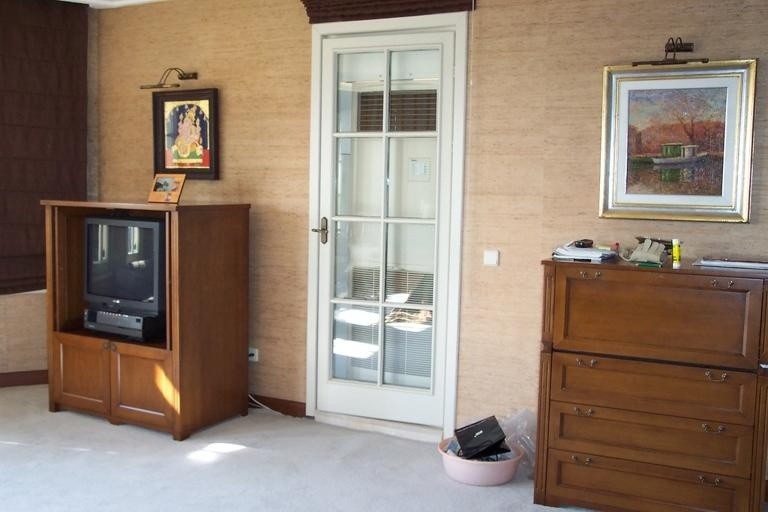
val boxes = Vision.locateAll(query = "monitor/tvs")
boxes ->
[81,216,166,317]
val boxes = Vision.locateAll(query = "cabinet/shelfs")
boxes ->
[533,243,767,512]
[38,197,251,440]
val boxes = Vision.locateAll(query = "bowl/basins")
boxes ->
[437,436,525,487]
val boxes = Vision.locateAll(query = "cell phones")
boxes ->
[574,238,594,247]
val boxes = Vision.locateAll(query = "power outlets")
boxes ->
[247,347,260,365]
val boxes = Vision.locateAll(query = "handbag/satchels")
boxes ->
[454,415,511,459]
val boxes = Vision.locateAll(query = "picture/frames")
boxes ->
[600,57,758,225]
[151,88,219,182]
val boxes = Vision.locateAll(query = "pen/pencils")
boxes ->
[635,262,661,267]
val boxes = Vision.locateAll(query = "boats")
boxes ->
[631,142,708,171]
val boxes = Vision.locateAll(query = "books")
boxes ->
[551,246,617,261]
[700,257,768,270]
